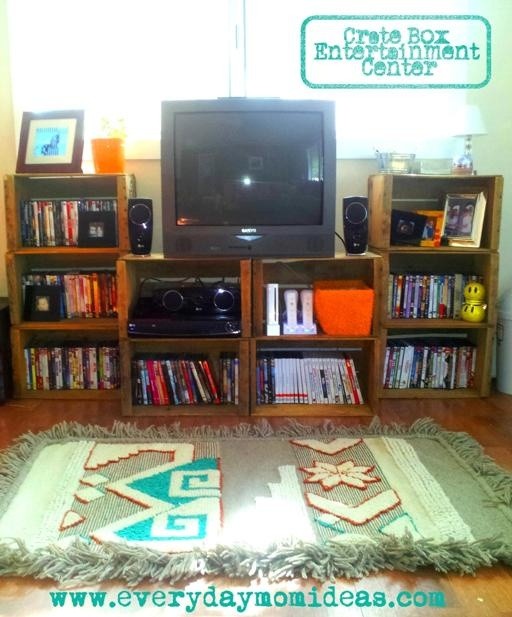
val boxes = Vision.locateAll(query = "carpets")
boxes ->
[0,415,512,590]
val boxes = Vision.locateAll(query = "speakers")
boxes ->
[343,196,369,256]
[153,287,241,316]
[128,199,153,257]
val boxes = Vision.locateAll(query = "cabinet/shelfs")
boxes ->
[367,174,504,399]
[117,250,380,417]
[3,175,136,401]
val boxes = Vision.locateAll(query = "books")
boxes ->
[130,350,367,407]
[17,196,120,391]
[383,267,483,392]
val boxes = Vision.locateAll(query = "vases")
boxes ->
[90,138,126,174]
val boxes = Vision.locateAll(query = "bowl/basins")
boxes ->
[376,152,416,174]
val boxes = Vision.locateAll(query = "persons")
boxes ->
[426,220,436,239]
[459,205,473,235]
[446,208,458,235]
[398,221,413,239]
[42,132,62,156]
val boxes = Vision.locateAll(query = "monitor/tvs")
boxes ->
[161,99,336,258]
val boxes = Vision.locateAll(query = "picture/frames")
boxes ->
[15,108,85,174]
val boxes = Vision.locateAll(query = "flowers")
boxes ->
[97,115,129,139]
